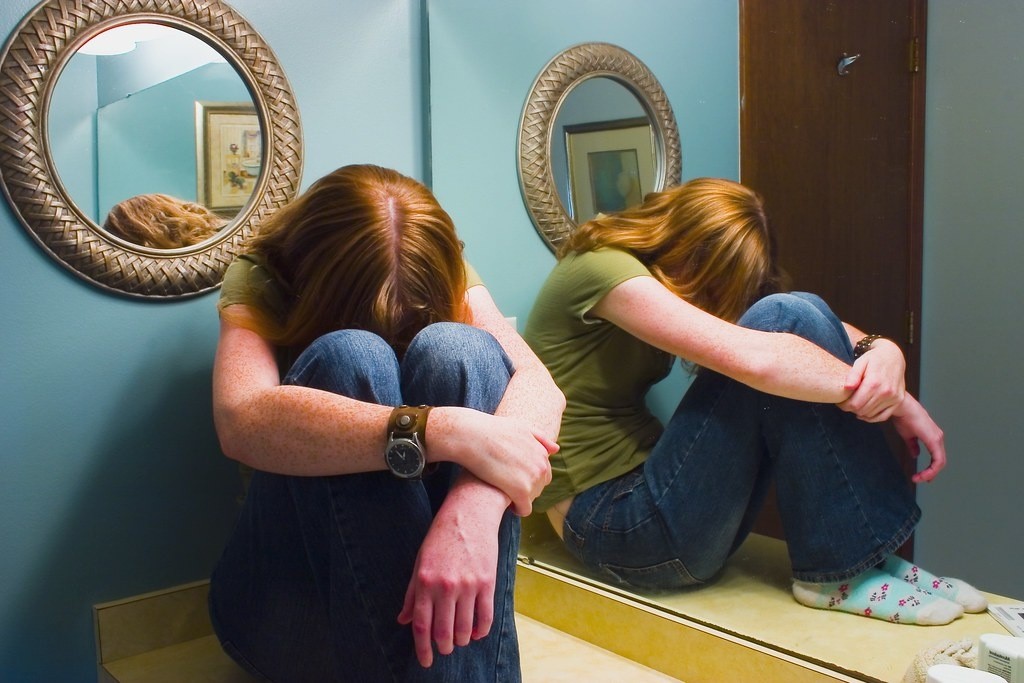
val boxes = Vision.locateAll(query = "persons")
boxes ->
[200,164,566,683]
[524,178,986,625]
[102,193,229,250]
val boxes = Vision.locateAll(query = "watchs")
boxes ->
[385,404,432,478]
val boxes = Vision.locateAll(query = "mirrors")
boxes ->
[516,43,684,256]
[422,0,1024,683]
[0,0,306,301]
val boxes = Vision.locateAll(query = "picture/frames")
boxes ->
[561,117,657,225]
[195,100,263,219]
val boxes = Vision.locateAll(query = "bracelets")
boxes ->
[854,333,882,356]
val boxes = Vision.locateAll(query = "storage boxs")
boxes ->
[985,604,1024,637]
